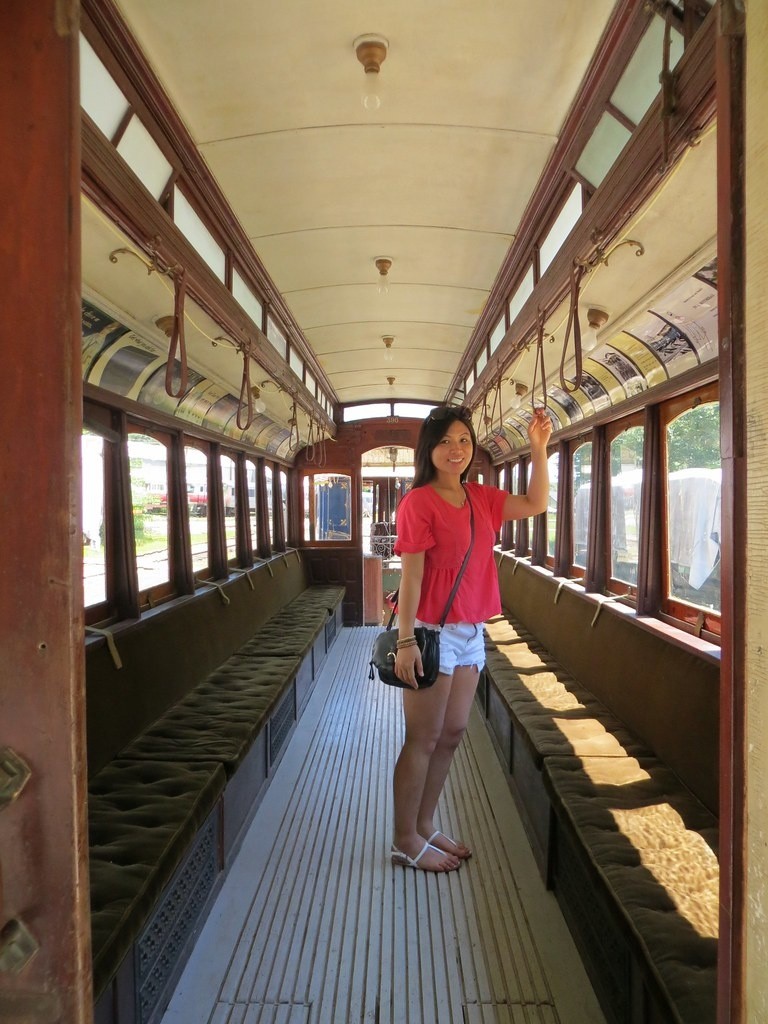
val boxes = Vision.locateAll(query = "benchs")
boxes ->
[83,552,347,1024]
[476,546,722,1024]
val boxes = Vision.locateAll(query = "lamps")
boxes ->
[372,256,393,297]
[387,376,395,395]
[476,417,492,441]
[287,417,300,437]
[394,478,400,490]
[381,335,396,363]
[389,449,398,462]
[510,383,529,408]
[353,33,389,112]
[580,309,610,351]
[249,384,266,413]
[152,312,181,359]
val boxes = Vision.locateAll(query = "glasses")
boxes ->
[426,406,472,424]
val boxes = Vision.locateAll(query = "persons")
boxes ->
[391,408,552,872]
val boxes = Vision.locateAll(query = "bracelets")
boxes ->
[396,636,417,649]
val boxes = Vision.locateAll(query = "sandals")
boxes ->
[417,831,472,859]
[391,842,462,871]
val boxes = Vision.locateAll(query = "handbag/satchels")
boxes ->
[369,627,441,689]
